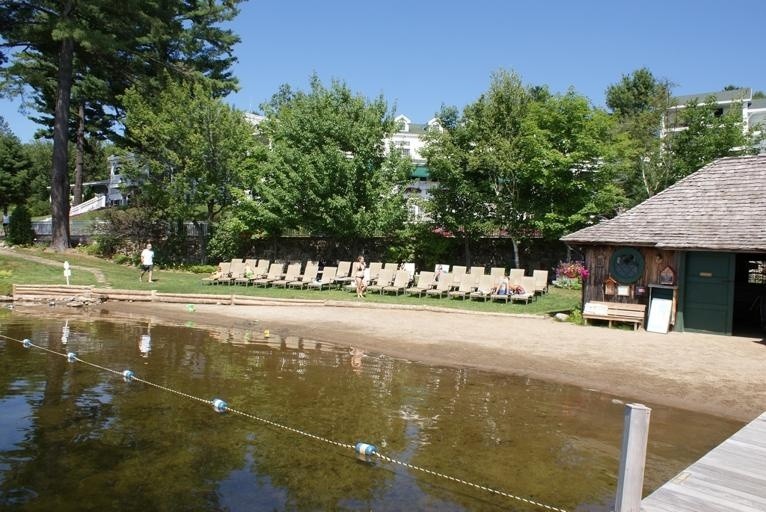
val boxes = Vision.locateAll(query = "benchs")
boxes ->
[582,298,648,331]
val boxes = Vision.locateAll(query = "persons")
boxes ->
[354,255,372,299]
[399,264,407,272]
[493,271,510,295]
[432,266,445,282]
[2,209,9,238]
[138,324,152,357]
[139,242,155,282]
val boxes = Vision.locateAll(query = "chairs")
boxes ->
[406,266,548,305]
[204,259,337,291]
[334,262,415,296]
